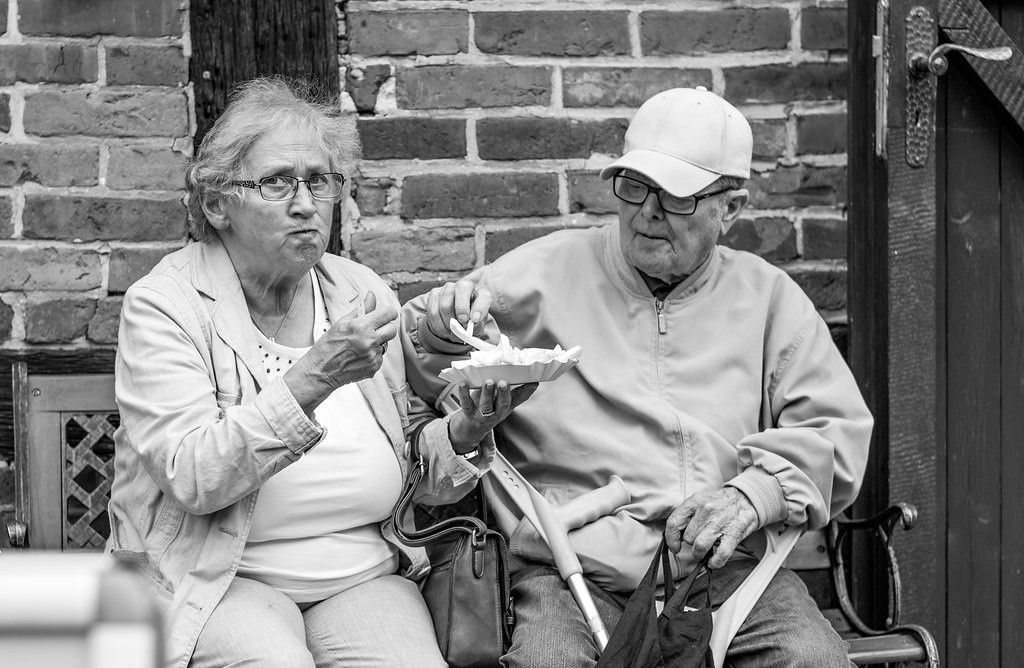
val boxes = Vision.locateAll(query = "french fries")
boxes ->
[449,317,582,366]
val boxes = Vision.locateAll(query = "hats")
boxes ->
[599,85,754,197]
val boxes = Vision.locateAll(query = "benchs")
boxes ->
[8,362,941,668]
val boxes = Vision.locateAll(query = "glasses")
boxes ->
[217,173,346,202]
[612,169,732,215]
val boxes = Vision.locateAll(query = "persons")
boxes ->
[94,74,539,667]
[386,84,875,668]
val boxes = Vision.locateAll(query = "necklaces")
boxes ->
[251,281,302,342]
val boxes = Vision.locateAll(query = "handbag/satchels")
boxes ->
[391,420,515,668]
[594,535,716,668]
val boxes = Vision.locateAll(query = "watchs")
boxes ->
[459,447,480,460]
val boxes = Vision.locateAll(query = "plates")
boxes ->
[438,347,580,389]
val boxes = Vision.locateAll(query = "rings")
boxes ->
[381,343,386,354]
[478,406,495,418]
